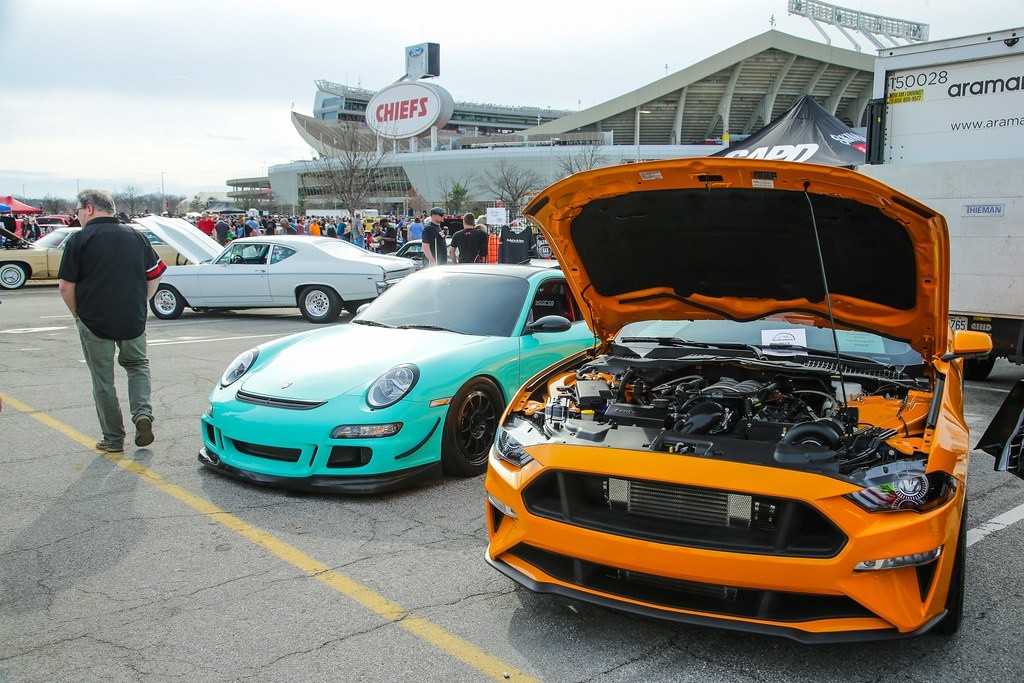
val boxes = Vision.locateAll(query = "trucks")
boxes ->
[837,24,1024,382]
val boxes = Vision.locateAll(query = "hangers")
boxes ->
[502,214,531,231]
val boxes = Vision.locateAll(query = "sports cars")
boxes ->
[195,261,604,498]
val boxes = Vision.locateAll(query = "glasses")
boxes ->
[435,214,444,217]
[73,207,86,216]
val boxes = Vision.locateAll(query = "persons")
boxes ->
[129,208,149,221]
[40,208,47,217]
[56,190,167,452]
[22,213,40,242]
[421,207,448,267]
[390,210,431,260]
[62,211,76,222]
[450,213,488,265]
[197,211,397,254]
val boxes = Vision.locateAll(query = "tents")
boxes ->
[0,195,43,218]
[0,204,13,217]
[202,203,246,237]
[710,94,867,168]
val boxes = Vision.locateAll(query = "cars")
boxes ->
[35,214,81,246]
[386,237,461,268]
[0,224,193,290]
[130,214,420,321]
[485,154,995,647]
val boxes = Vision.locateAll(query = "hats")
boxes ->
[378,219,387,225]
[430,207,446,214]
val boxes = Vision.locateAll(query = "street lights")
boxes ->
[161,171,167,212]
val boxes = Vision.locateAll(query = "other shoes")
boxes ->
[96,440,124,452]
[134,415,154,447]
